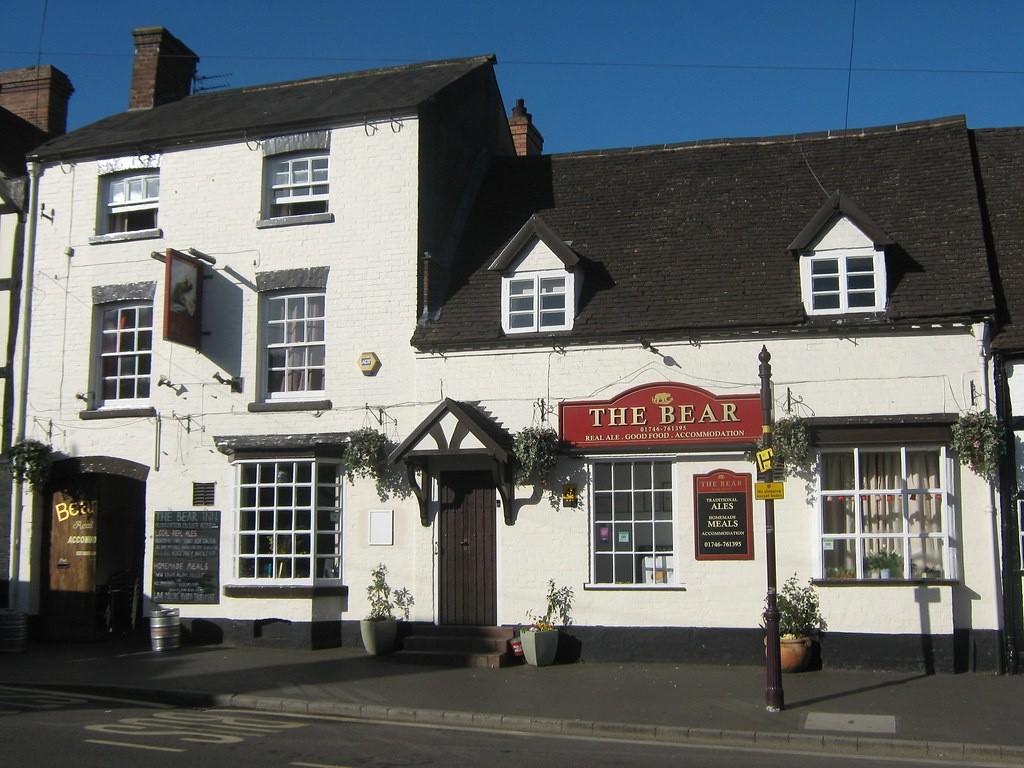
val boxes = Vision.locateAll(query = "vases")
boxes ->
[880,568,890,579]
[841,573,854,578]
[871,567,880,578]
[655,570,667,583]
[921,571,938,579]
[829,572,839,577]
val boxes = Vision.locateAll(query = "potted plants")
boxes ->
[519,579,565,667]
[759,573,821,674]
[360,563,415,656]
[8,439,49,495]
[511,425,562,487]
[744,415,812,481]
[343,427,388,502]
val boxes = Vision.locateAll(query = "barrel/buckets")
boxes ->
[0,608,27,654]
[151,608,181,653]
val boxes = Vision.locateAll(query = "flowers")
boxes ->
[948,409,1008,475]
